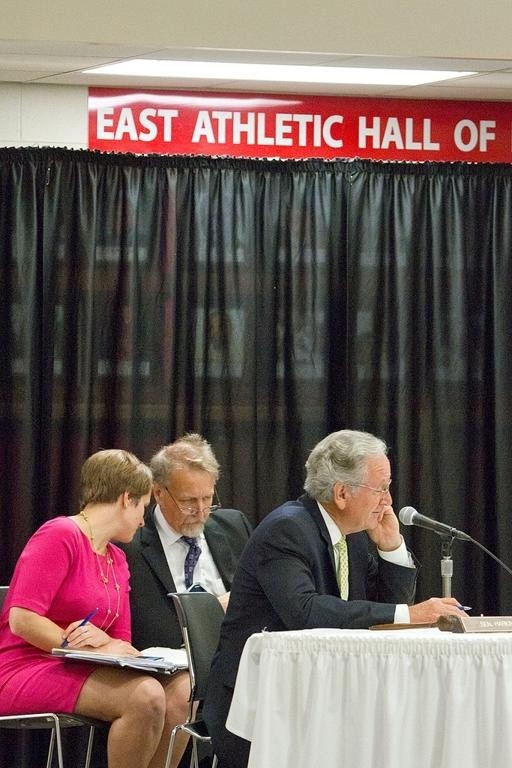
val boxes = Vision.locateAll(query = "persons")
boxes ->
[0,449,199,768]
[123,433,255,768]
[202,429,471,768]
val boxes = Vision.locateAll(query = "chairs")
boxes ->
[165,592,226,768]
[2,588,96,767]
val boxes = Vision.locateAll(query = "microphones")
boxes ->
[398,506,471,541]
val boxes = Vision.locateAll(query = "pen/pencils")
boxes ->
[60,608,99,649]
[455,606,473,611]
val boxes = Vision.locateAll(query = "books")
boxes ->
[52,647,189,676]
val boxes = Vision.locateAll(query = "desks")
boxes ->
[256,616,511,768]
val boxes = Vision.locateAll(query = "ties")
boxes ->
[181,536,201,589]
[335,536,350,601]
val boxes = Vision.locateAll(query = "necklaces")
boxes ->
[79,509,121,631]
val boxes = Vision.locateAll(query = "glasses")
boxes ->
[163,486,222,515]
[346,479,392,501]
